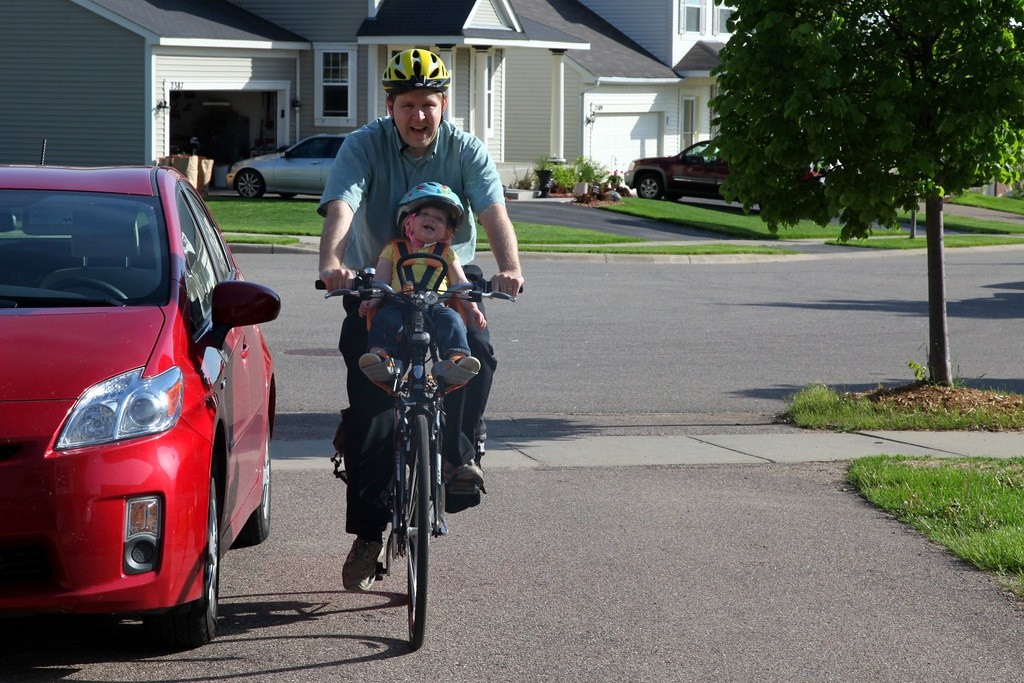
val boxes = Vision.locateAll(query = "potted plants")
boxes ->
[513,152,603,198]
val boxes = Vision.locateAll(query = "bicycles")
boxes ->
[314,265,523,652]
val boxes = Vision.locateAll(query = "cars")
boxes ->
[228,133,350,199]
[1,160,280,653]
[624,139,827,208]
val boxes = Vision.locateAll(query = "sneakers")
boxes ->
[444,462,484,517]
[435,354,481,397]
[360,350,402,397]
[340,537,385,593]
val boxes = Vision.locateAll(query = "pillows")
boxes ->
[70,203,144,259]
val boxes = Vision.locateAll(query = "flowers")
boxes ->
[607,169,627,184]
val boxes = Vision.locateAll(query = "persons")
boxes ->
[317,50,523,594]
[605,170,623,190]
[358,181,481,397]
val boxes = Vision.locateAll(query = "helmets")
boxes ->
[396,182,464,234]
[381,48,451,93]
